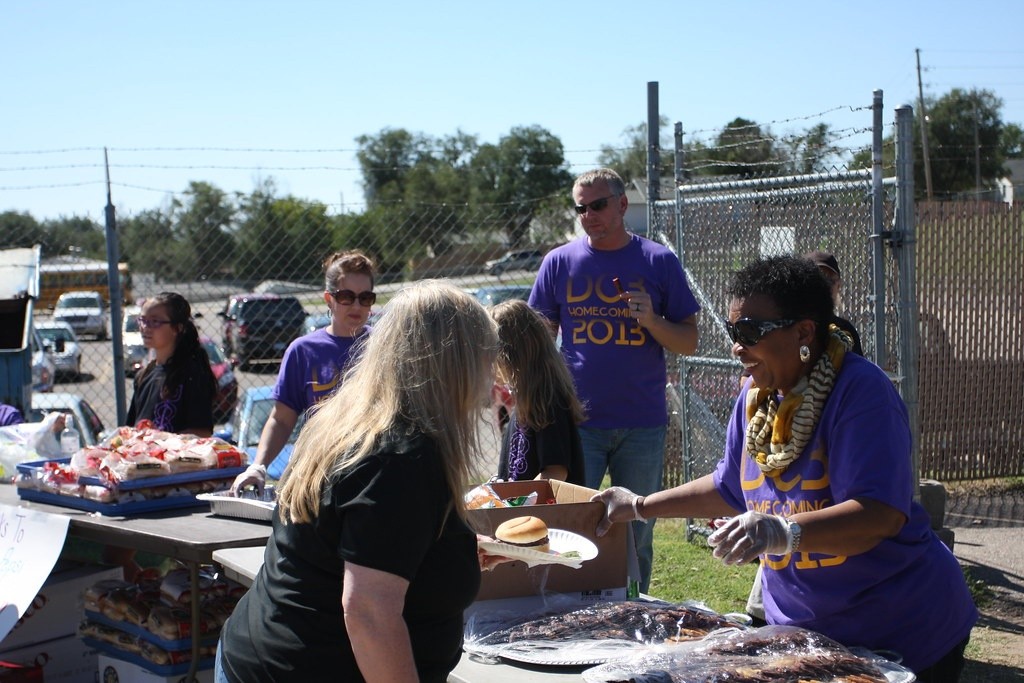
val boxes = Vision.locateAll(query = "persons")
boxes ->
[0,402,66,446]
[123,292,214,436]
[590,252,977,683]
[808,251,864,358]
[229,252,378,498]
[527,167,703,594]
[215,277,498,683]
[481,295,587,487]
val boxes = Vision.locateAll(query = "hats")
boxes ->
[806,251,840,276]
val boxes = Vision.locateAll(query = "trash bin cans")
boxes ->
[0,244,42,417]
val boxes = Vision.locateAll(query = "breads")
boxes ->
[77,564,247,668]
[14,428,249,504]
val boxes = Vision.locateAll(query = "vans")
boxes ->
[216,294,310,371]
[123,305,155,376]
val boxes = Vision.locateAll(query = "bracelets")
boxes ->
[637,496,645,517]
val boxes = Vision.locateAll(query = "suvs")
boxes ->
[484,249,546,279]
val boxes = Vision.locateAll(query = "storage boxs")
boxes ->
[463,478,644,628]
[0,556,218,683]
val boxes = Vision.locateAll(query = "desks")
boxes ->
[210,545,916,683]
[0,483,273,683]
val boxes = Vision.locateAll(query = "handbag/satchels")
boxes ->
[0,412,63,483]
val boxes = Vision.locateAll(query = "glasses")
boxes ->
[138,318,174,329]
[725,317,819,346]
[576,194,618,213]
[330,289,376,306]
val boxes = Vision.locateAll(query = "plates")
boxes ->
[477,528,599,563]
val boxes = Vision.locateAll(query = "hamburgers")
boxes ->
[495,516,550,553]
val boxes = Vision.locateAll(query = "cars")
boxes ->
[202,339,243,419]
[53,290,110,339]
[34,320,87,375]
[473,286,533,307]
[31,329,57,392]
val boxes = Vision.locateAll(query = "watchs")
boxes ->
[785,516,802,553]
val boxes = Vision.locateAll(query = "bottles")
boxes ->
[61,414,80,458]
